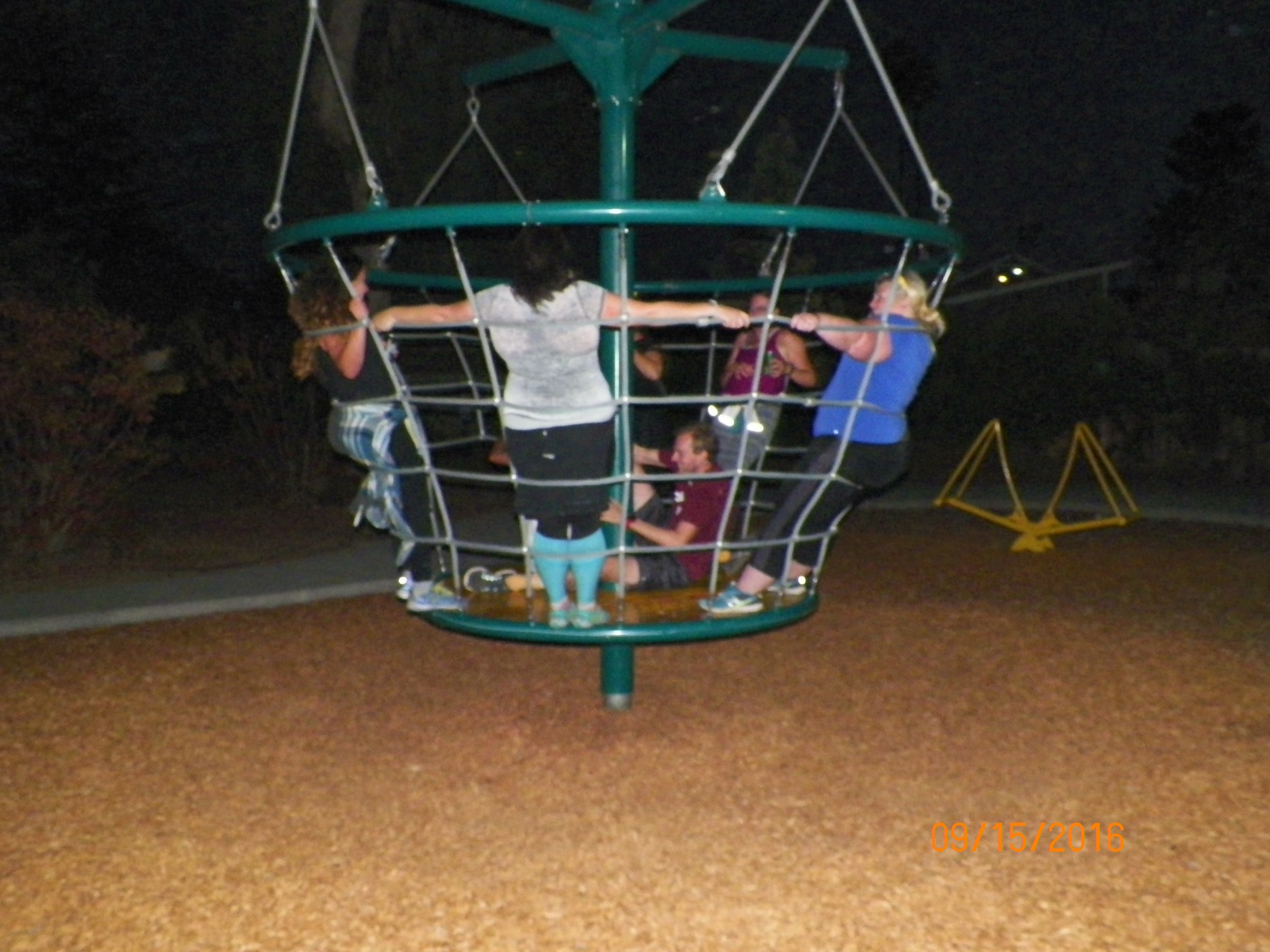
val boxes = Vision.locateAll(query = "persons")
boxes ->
[462,419,730,594]
[374,225,747,628]
[698,265,944,614]
[289,255,466,614]
[626,330,676,446]
[704,292,817,488]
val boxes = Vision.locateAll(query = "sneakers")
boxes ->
[769,579,806,596]
[697,583,764,613]
[398,580,433,600]
[406,590,470,612]
[463,566,517,594]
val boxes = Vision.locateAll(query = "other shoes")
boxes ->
[548,604,575,628]
[574,604,608,628]
